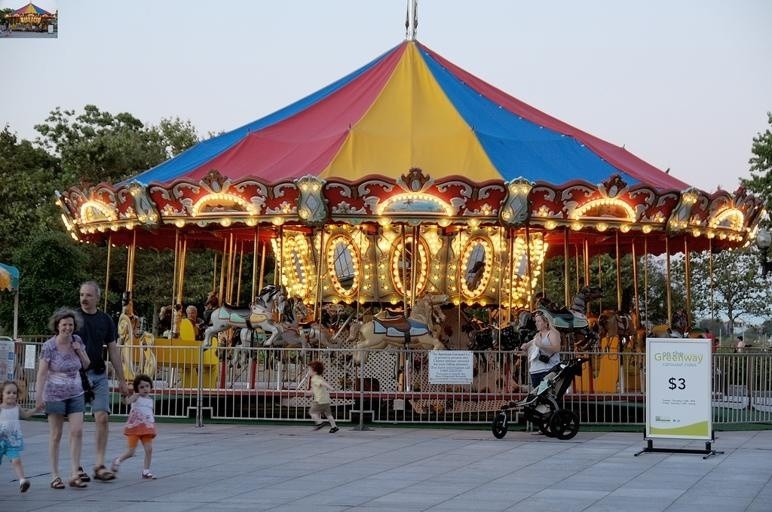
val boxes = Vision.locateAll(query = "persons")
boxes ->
[112,375,157,480]
[106,285,752,436]
[36,307,91,489]
[106,284,753,436]
[0,381,43,493]
[75,281,128,482]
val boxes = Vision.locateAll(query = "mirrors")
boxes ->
[455,234,495,301]
[389,231,432,299]
[283,233,312,298]
[513,247,530,292]
[324,231,364,299]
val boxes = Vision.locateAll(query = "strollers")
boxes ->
[491,351,589,439]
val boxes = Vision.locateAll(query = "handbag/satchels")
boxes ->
[79,368,96,404]
[540,355,549,363]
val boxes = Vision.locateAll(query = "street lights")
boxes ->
[758,224,772,280]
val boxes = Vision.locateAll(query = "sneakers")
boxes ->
[330,427,338,433]
[19,479,30,494]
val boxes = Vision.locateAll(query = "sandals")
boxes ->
[141,470,157,481]
[51,457,121,489]
